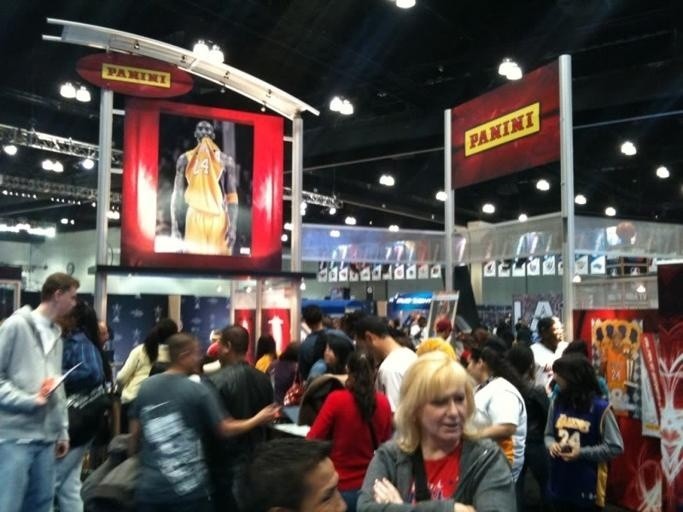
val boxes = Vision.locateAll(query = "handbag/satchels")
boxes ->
[283,383,304,408]
[63,363,116,432]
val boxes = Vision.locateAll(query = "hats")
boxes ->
[436,319,457,332]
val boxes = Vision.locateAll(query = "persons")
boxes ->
[169,120,238,256]
[0,273,624,512]
